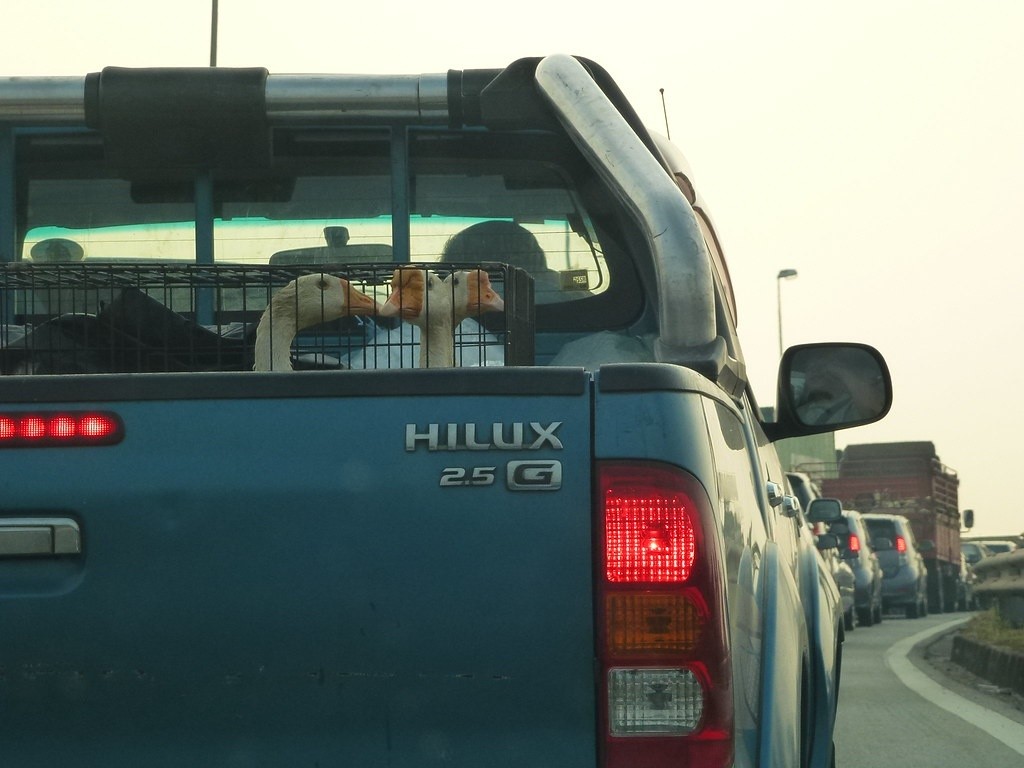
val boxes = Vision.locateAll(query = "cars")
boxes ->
[784,472,857,631]
[962,540,1017,611]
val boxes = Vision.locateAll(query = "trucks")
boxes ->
[819,440,974,612]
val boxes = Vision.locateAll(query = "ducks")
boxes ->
[252,265,503,372]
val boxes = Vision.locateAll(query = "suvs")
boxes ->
[838,509,884,626]
[862,513,936,618]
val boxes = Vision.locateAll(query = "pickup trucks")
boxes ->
[1,55,897,768]
